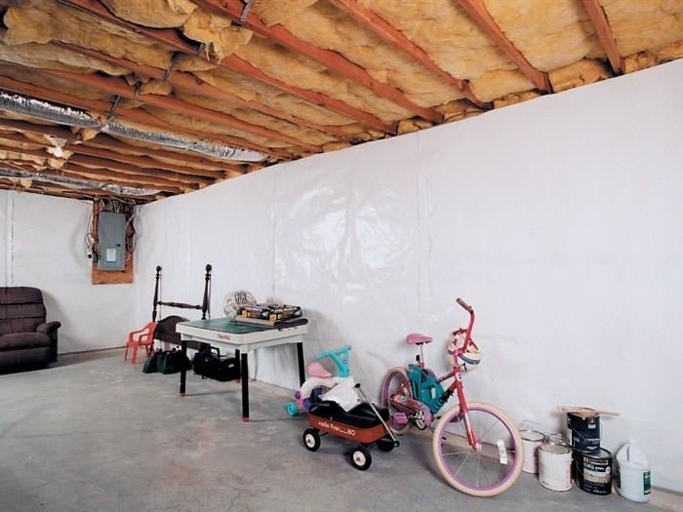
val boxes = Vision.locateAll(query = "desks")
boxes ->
[176,318,309,422]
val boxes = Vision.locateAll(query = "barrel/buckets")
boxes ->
[566,406,602,454]
[542,432,566,446]
[516,418,545,475]
[615,441,652,503]
[574,447,613,496]
[537,443,574,493]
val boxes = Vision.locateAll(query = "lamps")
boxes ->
[54,144,64,158]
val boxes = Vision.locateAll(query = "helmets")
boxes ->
[448,334,482,372]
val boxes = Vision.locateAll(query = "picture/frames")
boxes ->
[92,201,134,285]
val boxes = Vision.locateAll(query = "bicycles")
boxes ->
[373,298,525,498]
[285,346,353,415]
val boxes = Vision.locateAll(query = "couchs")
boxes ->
[0,287,61,375]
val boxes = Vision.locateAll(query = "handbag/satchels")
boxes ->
[143,350,192,374]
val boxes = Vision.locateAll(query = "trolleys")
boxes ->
[302,383,398,471]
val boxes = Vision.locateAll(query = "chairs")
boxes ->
[125,322,157,364]
